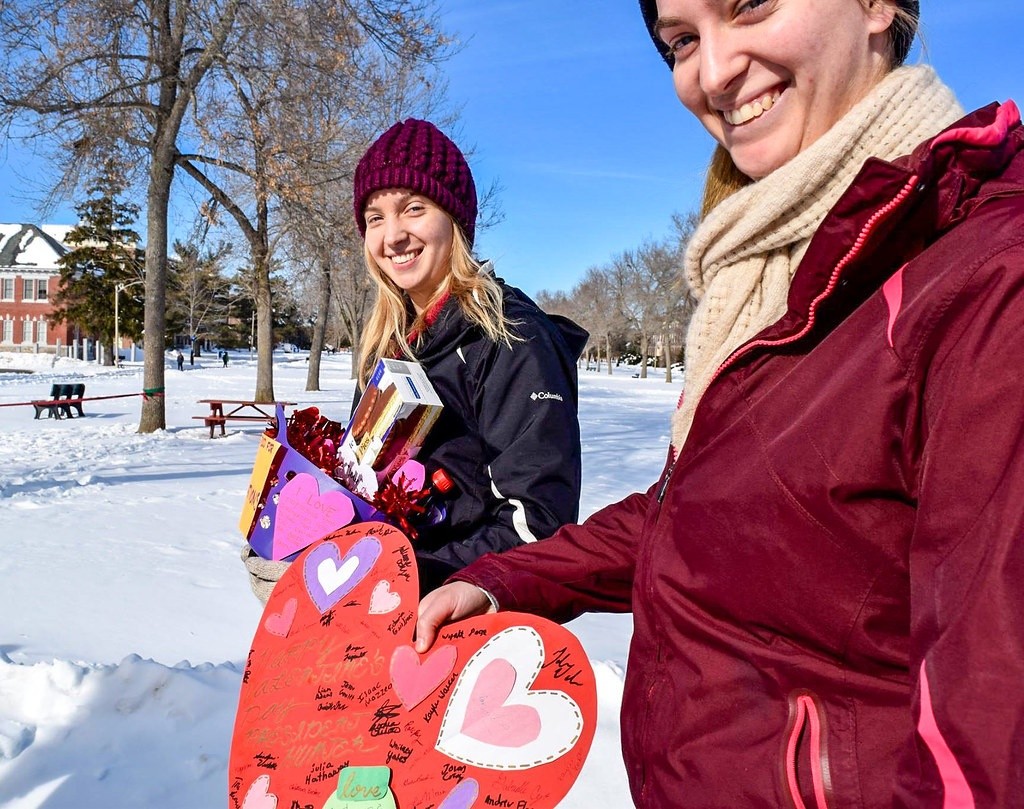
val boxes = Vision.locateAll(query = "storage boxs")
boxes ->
[339,358,444,486]
[239,433,386,561]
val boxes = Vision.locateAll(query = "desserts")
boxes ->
[405,403,443,449]
[352,385,383,445]
[366,382,402,441]
[372,418,408,471]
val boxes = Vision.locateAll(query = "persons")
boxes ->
[222,351,229,368]
[413,0,1023,809]
[176,351,184,372]
[241,120,589,626]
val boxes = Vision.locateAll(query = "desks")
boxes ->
[196,399,298,439]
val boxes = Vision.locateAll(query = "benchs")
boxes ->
[192,415,295,439]
[31,384,85,420]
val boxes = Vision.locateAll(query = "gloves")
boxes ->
[240,544,293,609]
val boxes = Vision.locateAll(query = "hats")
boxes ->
[353,118,478,253]
[639,0,919,73]
